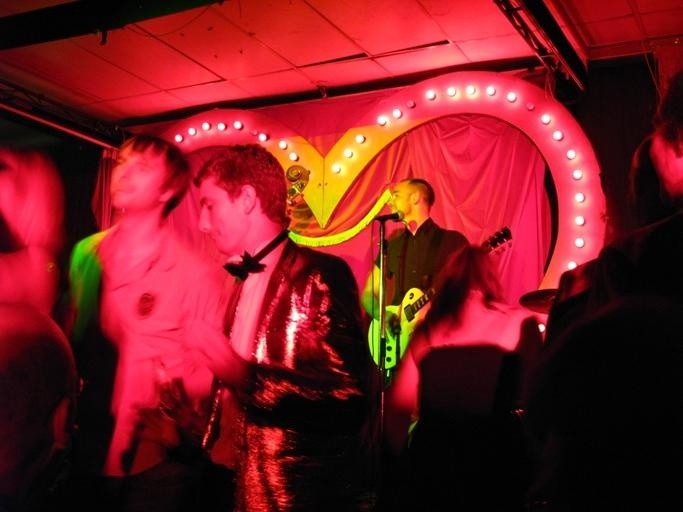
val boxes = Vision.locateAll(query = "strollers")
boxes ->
[223,250,267,281]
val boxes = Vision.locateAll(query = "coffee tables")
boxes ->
[519,289,558,314]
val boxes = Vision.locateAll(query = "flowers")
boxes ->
[375,211,404,222]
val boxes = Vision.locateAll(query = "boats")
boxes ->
[581,53,659,212]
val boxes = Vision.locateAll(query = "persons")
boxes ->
[0,144,89,508]
[371,244,540,425]
[60,132,241,472]
[152,142,385,504]
[231,296,500,299]
[353,173,472,368]
[593,69,683,302]
